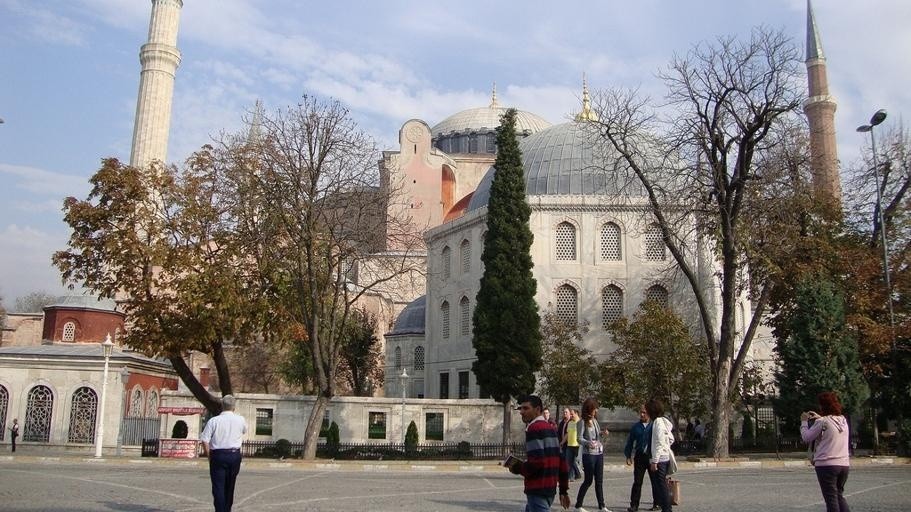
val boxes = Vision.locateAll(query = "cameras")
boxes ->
[808,413,813,420]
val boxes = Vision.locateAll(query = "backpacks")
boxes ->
[807,439,816,466]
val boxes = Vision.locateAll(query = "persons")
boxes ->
[200,393,247,512]
[508,393,572,512]
[543,407,558,431]
[644,399,675,512]
[559,409,582,482]
[7,419,19,452]
[573,398,612,512]
[623,403,662,512]
[556,406,569,458]
[798,390,851,512]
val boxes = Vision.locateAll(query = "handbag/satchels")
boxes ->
[667,449,678,475]
[667,477,680,505]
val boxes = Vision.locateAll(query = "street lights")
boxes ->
[855,110,906,460]
[93,333,114,460]
[399,368,409,445]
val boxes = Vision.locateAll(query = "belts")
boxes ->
[211,449,240,453]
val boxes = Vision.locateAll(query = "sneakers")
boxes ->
[574,506,588,512]
[597,507,612,512]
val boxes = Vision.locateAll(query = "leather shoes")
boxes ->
[649,505,660,511]
[628,506,638,511]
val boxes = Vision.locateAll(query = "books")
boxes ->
[499,455,524,469]
